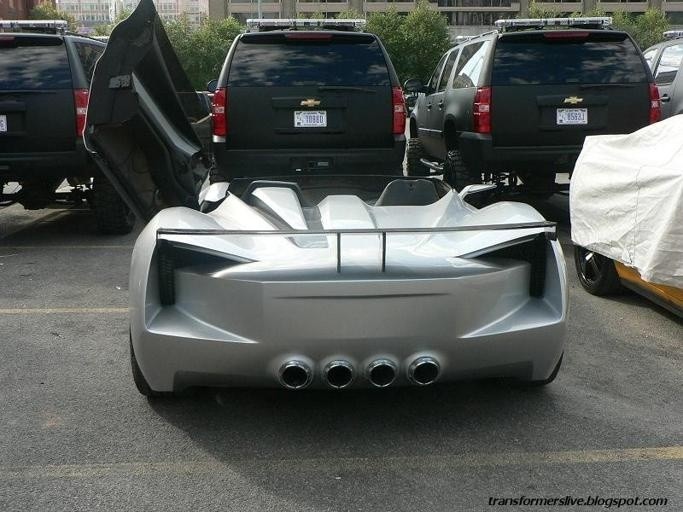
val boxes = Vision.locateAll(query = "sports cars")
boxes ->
[79,0,571,402]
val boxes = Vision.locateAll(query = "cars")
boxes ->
[563,114,682,322]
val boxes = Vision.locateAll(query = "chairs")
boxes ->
[371,177,441,208]
[240,178,307,208]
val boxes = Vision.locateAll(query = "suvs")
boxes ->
[402,15,661,212]
[206,16,416,195]
[0,19,140,235]
[180,89,218,172]
[641,28,682,124]
